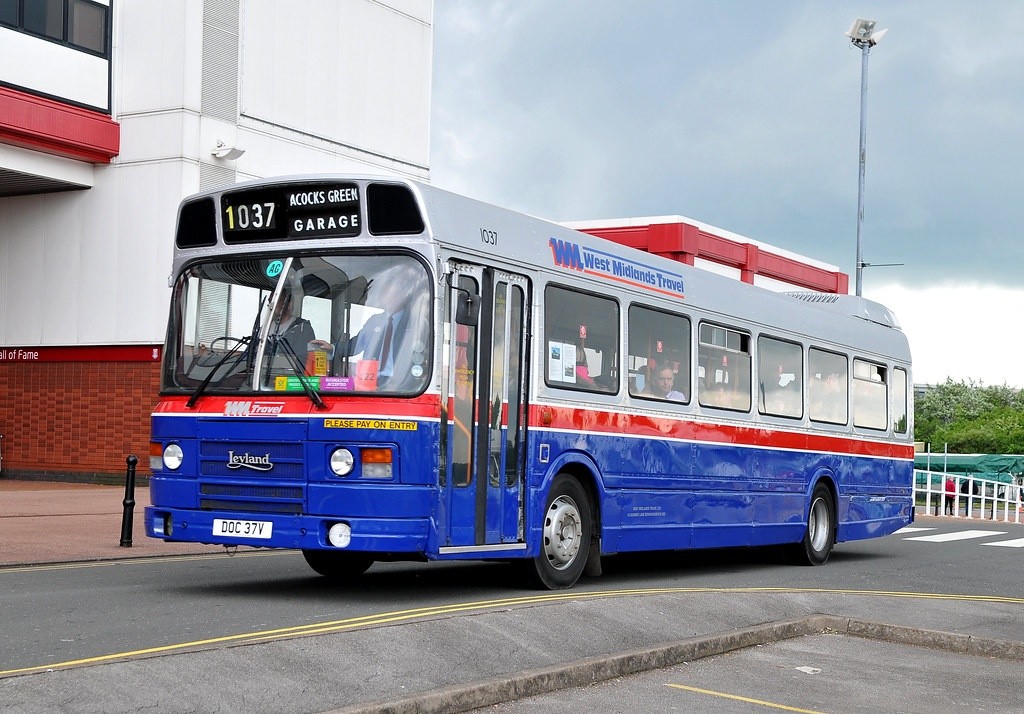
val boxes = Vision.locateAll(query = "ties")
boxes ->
[379,317,393,372]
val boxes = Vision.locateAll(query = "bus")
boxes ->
[144,174,915,591]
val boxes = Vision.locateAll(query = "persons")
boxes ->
[698,358,847,425]
[945,476,956,515]
[309,273,429,392]
[961,475,978,516]
[628,361,690,405]
[576,344,599,390]
[245,288,316,366]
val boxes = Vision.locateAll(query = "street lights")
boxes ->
[845,15,905,298]
